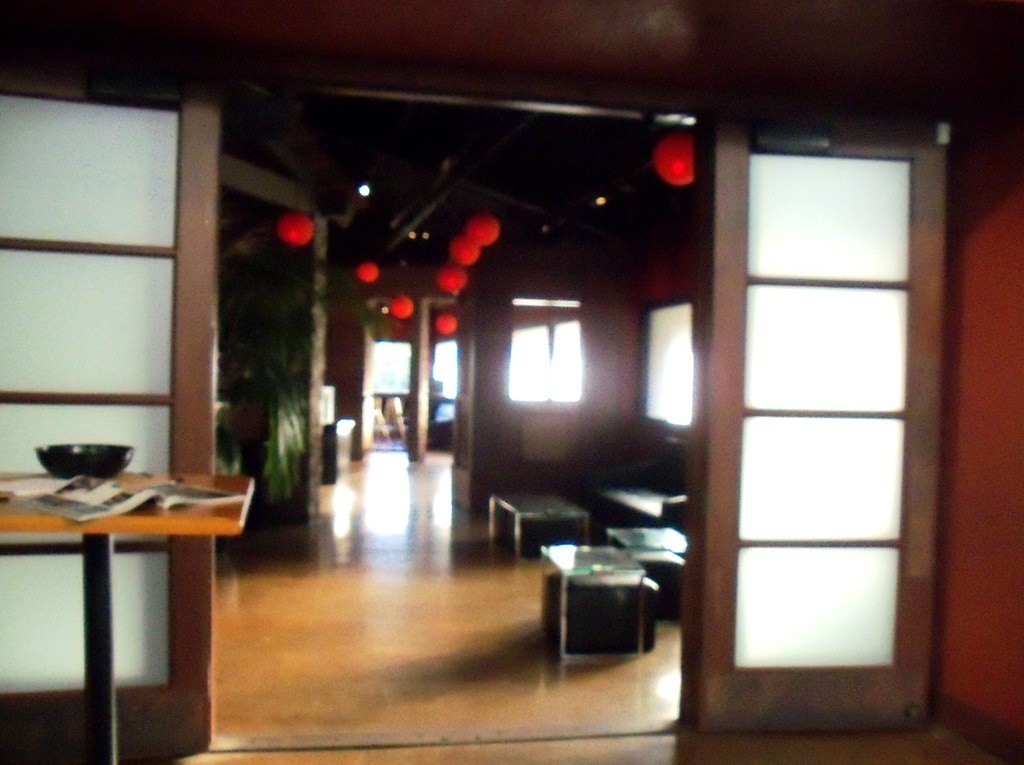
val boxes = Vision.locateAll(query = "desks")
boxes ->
[537,542,648,667]
[0,471,256,765]
[486,489,591,564]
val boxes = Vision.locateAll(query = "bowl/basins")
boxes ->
[35,445,134,479]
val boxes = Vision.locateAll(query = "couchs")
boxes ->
[584,436,689,530]
